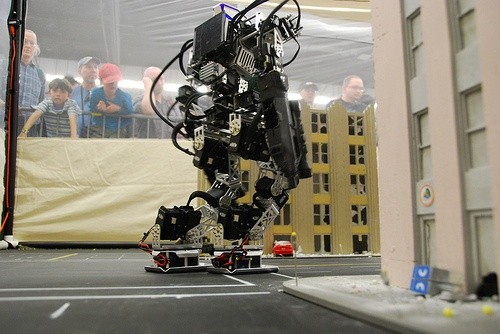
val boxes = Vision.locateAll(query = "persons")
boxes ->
[131,66,188,139]
[324,74,368,112]
[0,28,47,137]
[297,80,319,107]
[87,62,134,138]
[70,55,103,138]
[17,77,83,138]
[63,74,80,87]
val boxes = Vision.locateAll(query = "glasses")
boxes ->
[344,85,365,93]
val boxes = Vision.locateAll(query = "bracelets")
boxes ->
[20,128,29,134]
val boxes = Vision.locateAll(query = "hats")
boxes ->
[98,63,122,84]
[77,56,102,68]
[298,80,319,92]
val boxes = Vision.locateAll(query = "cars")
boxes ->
[273,241,293,257]
